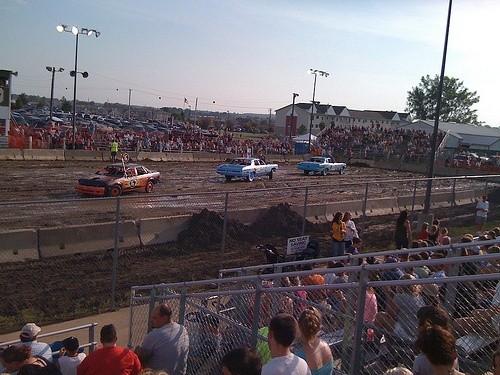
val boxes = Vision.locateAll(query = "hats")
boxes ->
[63,336,79,348]
[20,323,42,338]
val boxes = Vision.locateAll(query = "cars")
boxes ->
[216,157,281,182]
[74,162,162,199]
[298,156,348,175]
[10,104,244,139]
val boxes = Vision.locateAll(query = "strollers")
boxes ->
[291,239,325,273]
[255,243,289,276]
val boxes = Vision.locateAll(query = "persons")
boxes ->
[342,210,359,251]
[3,344,63,375]
[220,347,263,375]
[225,220,500,357]
[259,313,312,375]
[136,303,190,375]
[139,367,168,375]
[58,336,88,375]
[394,209,413,250]
[418,325,466,375]
[17,323,54,363]
[330,212,346,257]
[412,305,458,375]
[77,323,143,375]
[9,124,447,162]
[293,306,333,375]
[382,366,414,375]
[475,195,489,233]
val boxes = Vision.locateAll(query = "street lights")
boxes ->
[306,67,330,153]
[46,66,64,119]
[290,92,300,140]
[57,23,100,152]
